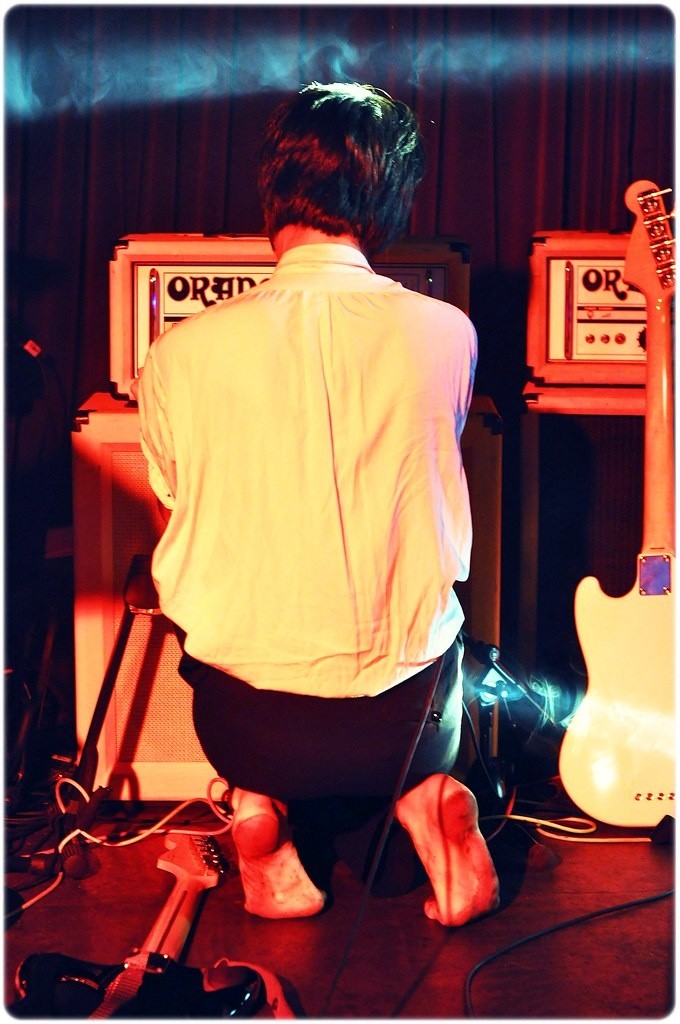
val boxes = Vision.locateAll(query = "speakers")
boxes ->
[71,394,507,799]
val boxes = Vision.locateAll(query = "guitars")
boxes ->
[3,821,297,1021]
[546,172,676,836]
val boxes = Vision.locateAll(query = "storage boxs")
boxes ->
[105,230,472,407]
[525,230,649,386]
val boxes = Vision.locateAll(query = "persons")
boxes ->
[132,79,502,927]
